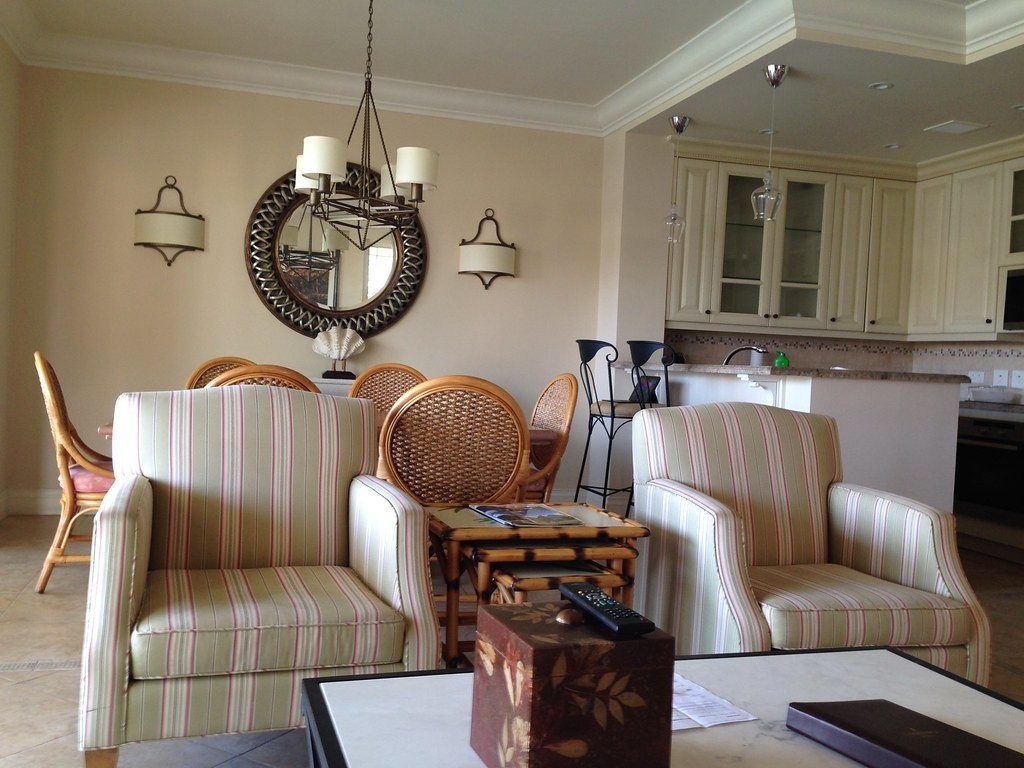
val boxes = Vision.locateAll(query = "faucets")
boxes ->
[722,345,768,365]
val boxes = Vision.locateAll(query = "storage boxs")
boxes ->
[470,602,675,768]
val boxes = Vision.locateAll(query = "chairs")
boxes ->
[30,349,579,609]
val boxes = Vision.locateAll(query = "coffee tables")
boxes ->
[426,498,652,671]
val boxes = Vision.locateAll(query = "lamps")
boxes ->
[132,176,206,263]
[751,61,790,219]
[458,206,517,289]
[664,116,691,244]
[295,1,441,249]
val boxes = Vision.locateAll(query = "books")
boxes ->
[469,503,585,527]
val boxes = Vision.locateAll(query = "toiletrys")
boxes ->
[774,348,789,367]
[750,341,764,366]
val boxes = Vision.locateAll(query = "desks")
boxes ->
[299,638,1024,768]
[95,411,554,445]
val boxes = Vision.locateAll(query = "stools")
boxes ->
[576,339,674,517]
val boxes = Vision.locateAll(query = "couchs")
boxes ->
[78,384,443,768]
[632,399,991,690]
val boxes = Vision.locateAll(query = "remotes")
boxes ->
[558,581,655,636]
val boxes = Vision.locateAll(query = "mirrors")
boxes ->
[243,161,427,343]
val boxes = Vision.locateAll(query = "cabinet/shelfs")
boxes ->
[665,157,1024,347]
